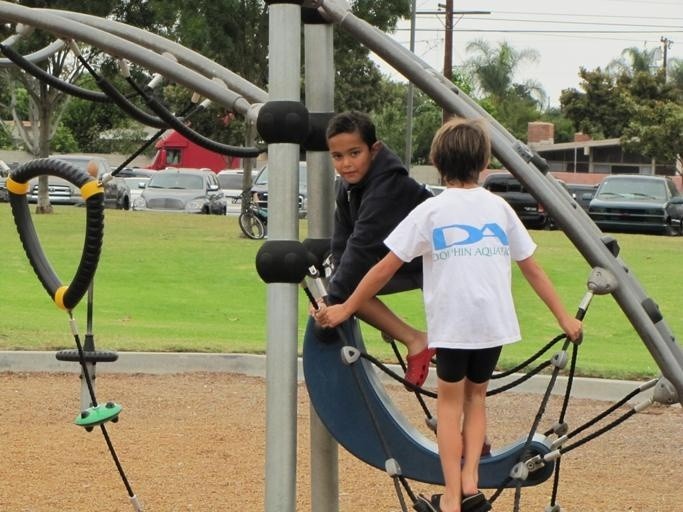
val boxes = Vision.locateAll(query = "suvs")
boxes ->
[133,167,228,216]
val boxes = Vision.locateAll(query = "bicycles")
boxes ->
[232,185,267,240]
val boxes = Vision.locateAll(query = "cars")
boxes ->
[26,155,131,212]
[217,169,258,205]
[249,162,310,219]
[429,173,683,236]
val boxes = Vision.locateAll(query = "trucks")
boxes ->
[144,112,240,175]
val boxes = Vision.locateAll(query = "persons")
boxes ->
[324,117,583,512]
[309,111,440,392]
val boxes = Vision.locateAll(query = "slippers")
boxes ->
[404,343,437,393]
[417,490,487,512]
